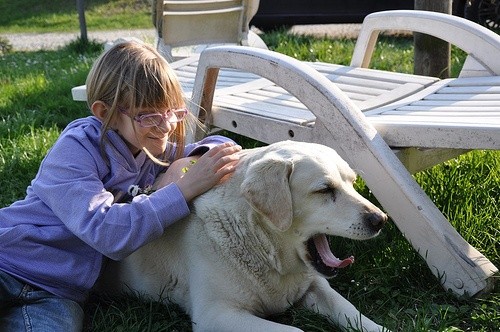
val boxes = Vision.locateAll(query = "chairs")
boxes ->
[153,0,260,64]
[71,9,500,303]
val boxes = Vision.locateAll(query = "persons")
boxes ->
[0,39,244,332]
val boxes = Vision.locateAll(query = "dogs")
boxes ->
[88,138,394,332]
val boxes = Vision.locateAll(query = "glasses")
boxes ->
[117,106,187,128]
